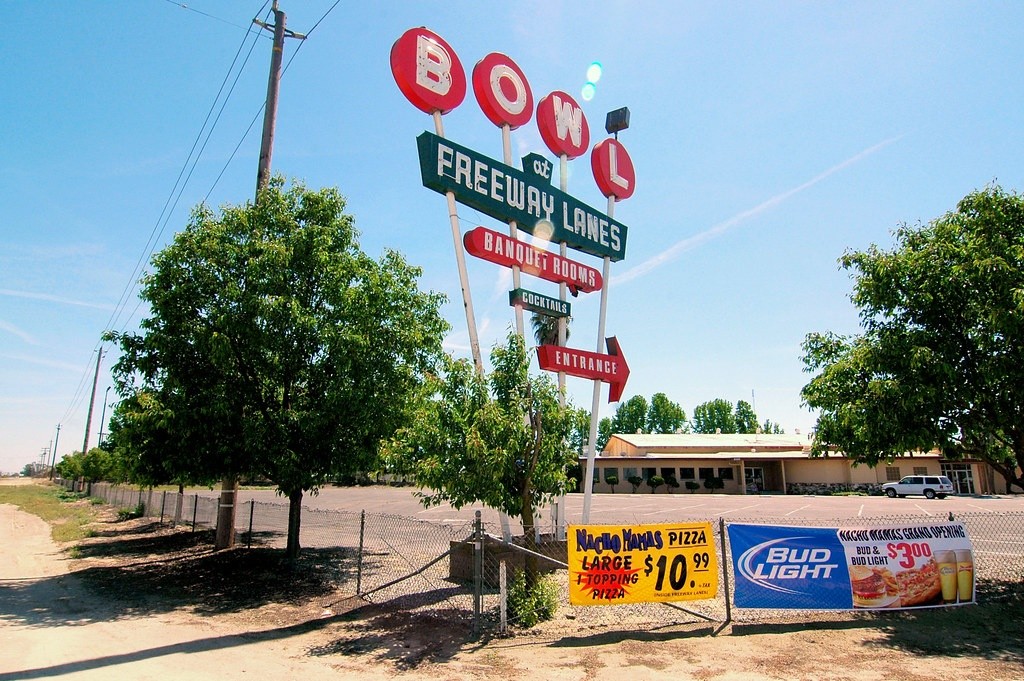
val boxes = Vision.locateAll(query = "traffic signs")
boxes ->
[537,336,631,403]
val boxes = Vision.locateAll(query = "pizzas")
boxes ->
[897,556,941,605]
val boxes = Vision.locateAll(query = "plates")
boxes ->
[852,594,899,608]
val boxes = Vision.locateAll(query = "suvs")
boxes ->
[881,475,957,499]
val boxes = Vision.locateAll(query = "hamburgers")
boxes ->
[851,565,888,604]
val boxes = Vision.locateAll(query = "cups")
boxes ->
[953,549,973,603]
[933,550,958,604]
